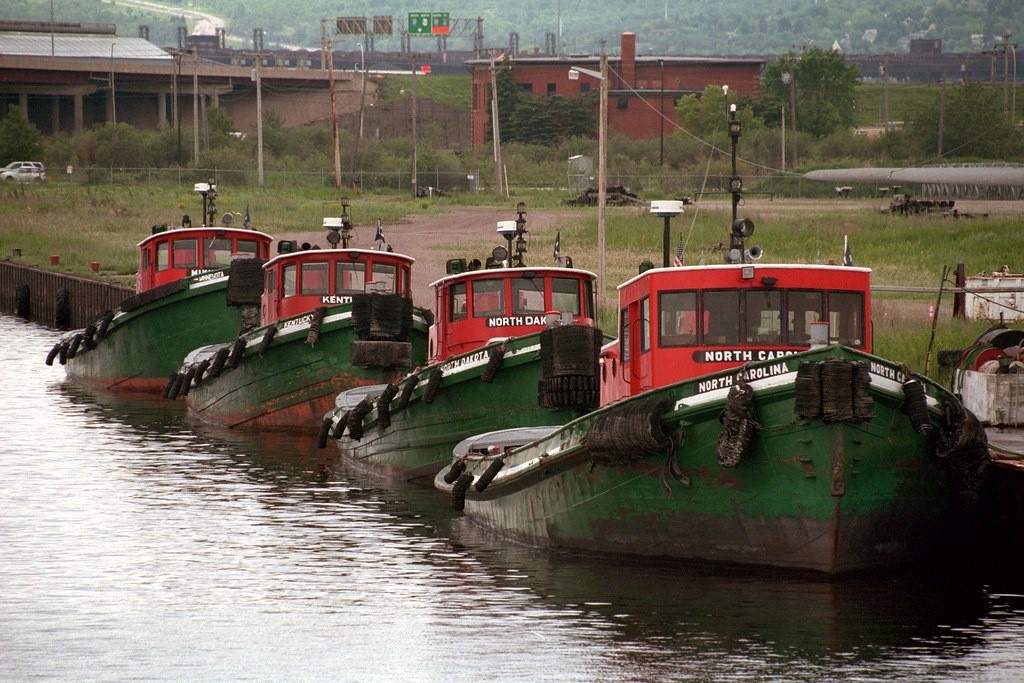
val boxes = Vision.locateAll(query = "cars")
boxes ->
[0,161,48,183]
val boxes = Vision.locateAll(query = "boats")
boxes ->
[45,176,353,394]
[934,263,1024,509]
[161,194,436,436]
[317,200,605,489]
[433,119,990,576]
